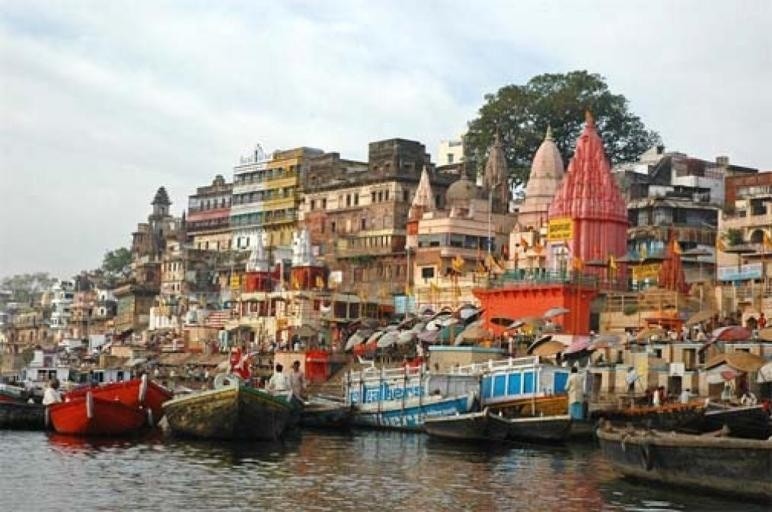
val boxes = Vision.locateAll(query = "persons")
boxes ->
[653,385,665,406]
[565,366,586,420]
[677,388,698,404]
[722,386,730,400]
[268,360,307,400]
[740,388,758,405]
[626,366,635,391]
[42,378,62,405]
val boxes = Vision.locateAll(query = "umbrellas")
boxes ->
[344,303,626,365]
[636,315,772,384]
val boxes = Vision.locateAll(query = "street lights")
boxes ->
[404,242,412,287]
[485,180,505,259]
